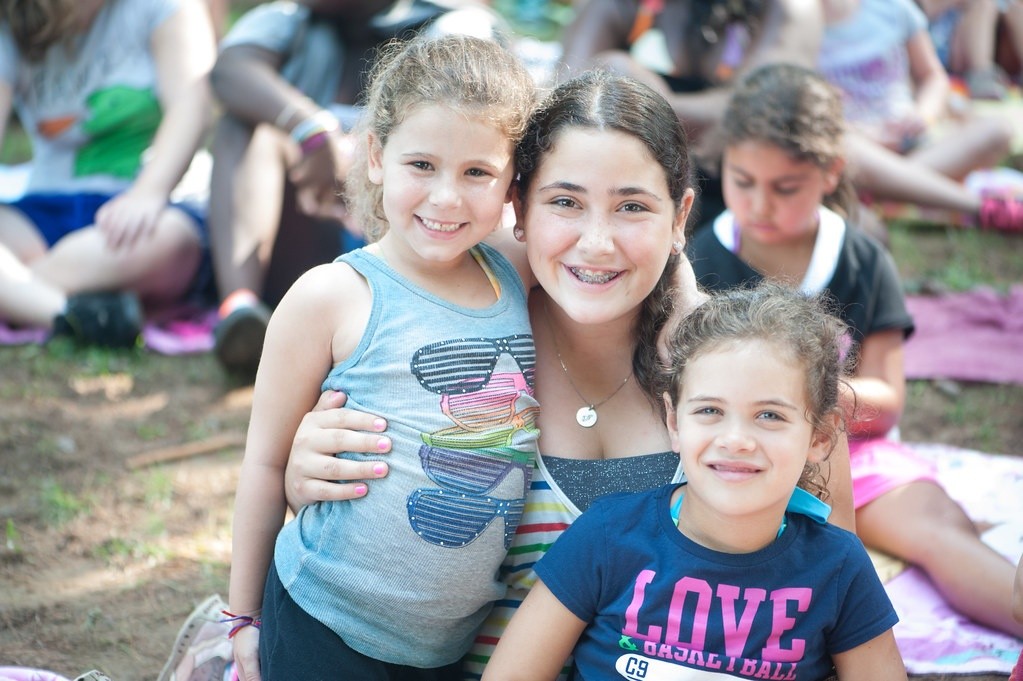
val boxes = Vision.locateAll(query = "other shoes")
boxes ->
[977,185,1023,235]
[156,594,234,681]
[53,287,141,347]
[214,292,270,377]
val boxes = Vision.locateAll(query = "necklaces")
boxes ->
[541,302,634,428]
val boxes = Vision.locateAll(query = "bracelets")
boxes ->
[288,109,335,141]
[274,96,316,128]
[298,122,342,160]
[218,608,262,639]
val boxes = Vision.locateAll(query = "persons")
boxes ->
[283,68,858,680]
[480,282,909,680]
[685,61,1023,681]
[0,0,1023,374]
[225,35,698,680]
